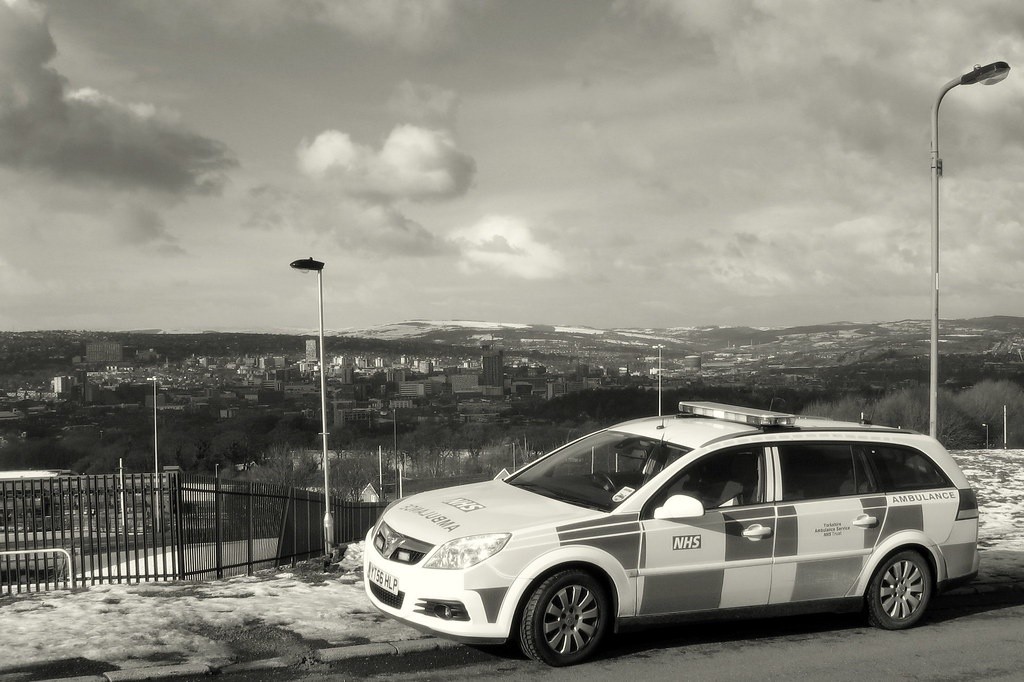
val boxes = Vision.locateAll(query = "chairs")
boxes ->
[697,454,761,507]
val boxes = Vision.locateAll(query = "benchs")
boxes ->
[785,453,871,496]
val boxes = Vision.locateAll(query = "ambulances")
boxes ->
[361,400,983,666]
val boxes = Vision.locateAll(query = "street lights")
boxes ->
[926,60,1013,439]
[291,255,335,558]
[146,376,161,532]
[653,344,665,418]
